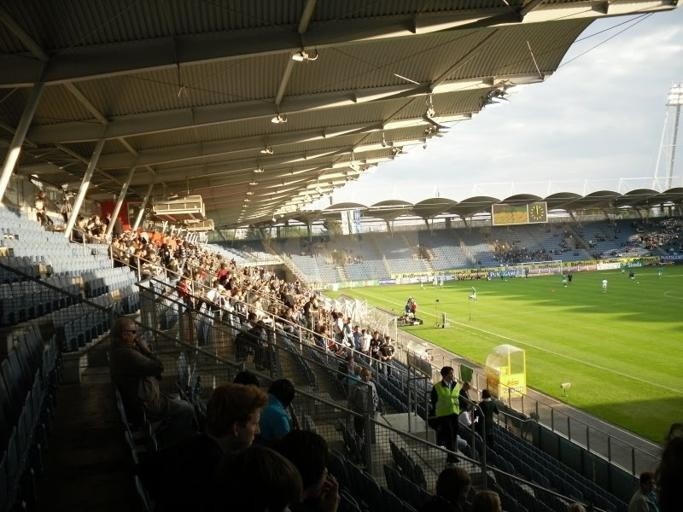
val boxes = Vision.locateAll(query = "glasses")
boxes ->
[124,329,138,334]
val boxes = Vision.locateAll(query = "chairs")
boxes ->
[0,201,631,511]
